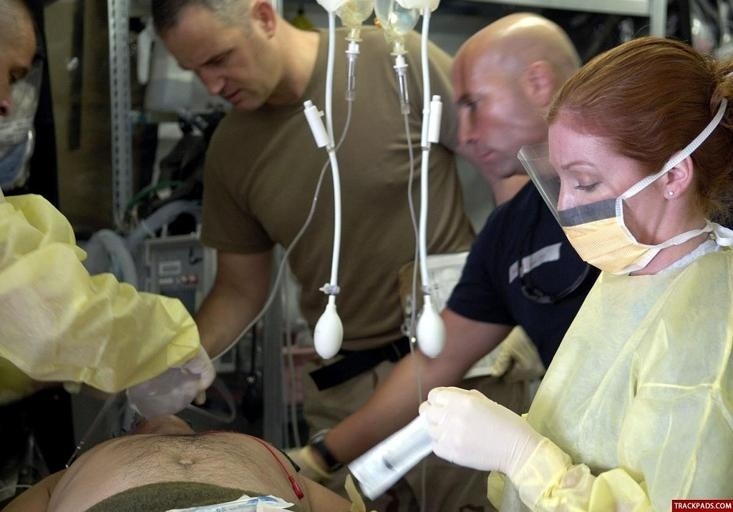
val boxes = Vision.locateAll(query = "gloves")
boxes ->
[417,385,572,512]
[181,344,216,405]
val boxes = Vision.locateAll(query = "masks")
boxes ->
[555,197,663,277]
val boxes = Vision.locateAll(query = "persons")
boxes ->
[1,413,368,512]
[0,0,216,414]
[284,12,599,485]
[147,1,536,512]
[418,36,733,512]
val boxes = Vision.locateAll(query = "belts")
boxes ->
[309,337,410,392]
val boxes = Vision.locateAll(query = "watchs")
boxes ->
[308,428,343,473]
